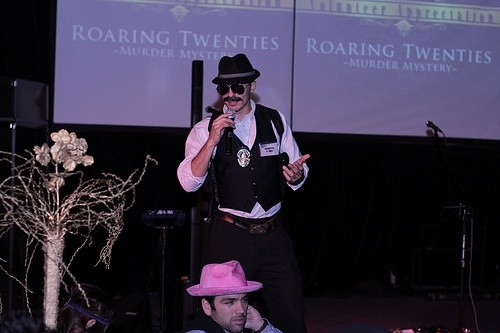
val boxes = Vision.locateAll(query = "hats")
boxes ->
[185,260,263,297]
[212,53,261,90]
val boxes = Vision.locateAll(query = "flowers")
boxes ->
[0,128,159,317]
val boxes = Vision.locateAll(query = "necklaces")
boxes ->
[233,117,252,168]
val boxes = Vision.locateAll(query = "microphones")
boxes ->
[425,120,443,132]
[225,108,236,156]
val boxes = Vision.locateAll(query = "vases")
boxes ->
[41,238,65,332]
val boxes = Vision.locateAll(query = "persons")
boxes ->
[176,53,310,333]
[56,283,116,333]
[185,260,283,333]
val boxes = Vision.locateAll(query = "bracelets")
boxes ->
[254,319,267,333]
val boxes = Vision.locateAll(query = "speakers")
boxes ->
[0,76,50,128]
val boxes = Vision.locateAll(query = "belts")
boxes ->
[216,213,282,235]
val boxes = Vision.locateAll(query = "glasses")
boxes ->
[215,84,251,94]
